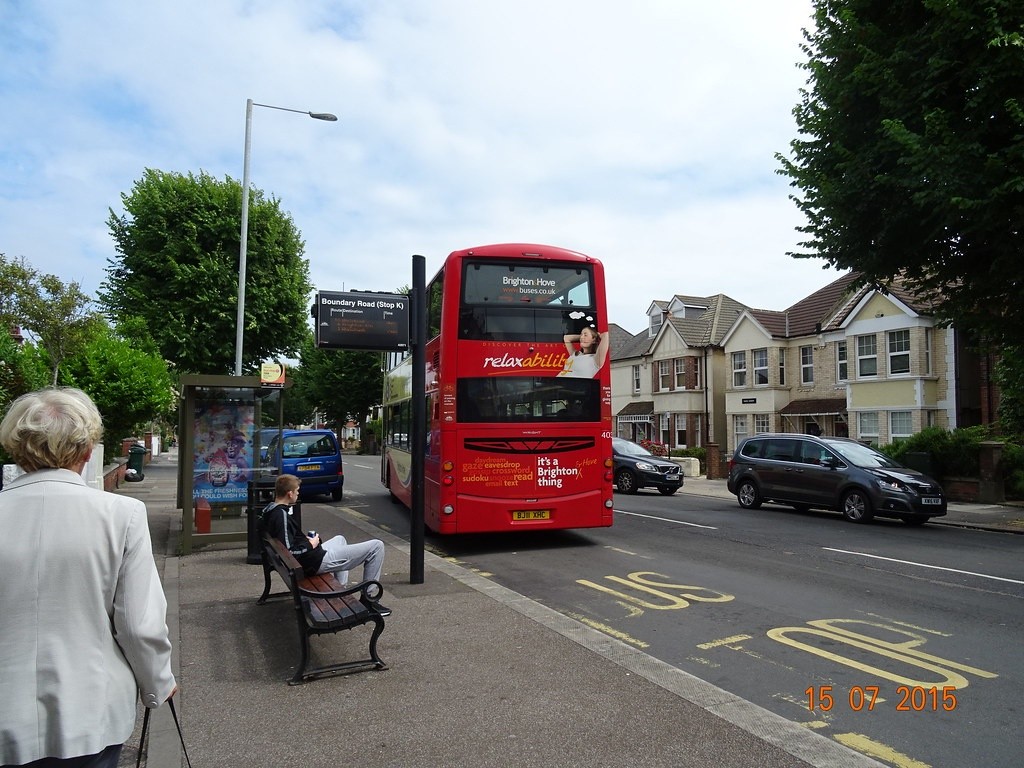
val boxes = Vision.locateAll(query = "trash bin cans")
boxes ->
[128,444,148,476]
[245,474,279,566]
[163,440,170,452]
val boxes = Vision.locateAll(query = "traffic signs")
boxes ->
[314,292,409,351]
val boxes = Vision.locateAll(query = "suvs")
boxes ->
[611,436,684,496]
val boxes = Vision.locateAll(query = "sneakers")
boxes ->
[359,600,391,616]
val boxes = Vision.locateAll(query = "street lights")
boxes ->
[236,100,339,393]
[667,411,671,459]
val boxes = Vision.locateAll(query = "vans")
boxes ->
[727,433,948,524]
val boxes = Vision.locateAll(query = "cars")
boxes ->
[267,432,344,501]
[260,429,296,463]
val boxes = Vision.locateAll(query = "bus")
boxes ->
[381,246,613,537]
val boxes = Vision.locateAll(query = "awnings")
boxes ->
[779,399,847,433]
[616,402,656,433]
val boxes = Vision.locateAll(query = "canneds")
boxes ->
[308,530,316,538]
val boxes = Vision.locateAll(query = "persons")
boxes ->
[172,436,176,449]
[261,473,393,616]
[0,388,178,768]
[556,326,609,378]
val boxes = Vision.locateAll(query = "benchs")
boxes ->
[256,515,389,686]
